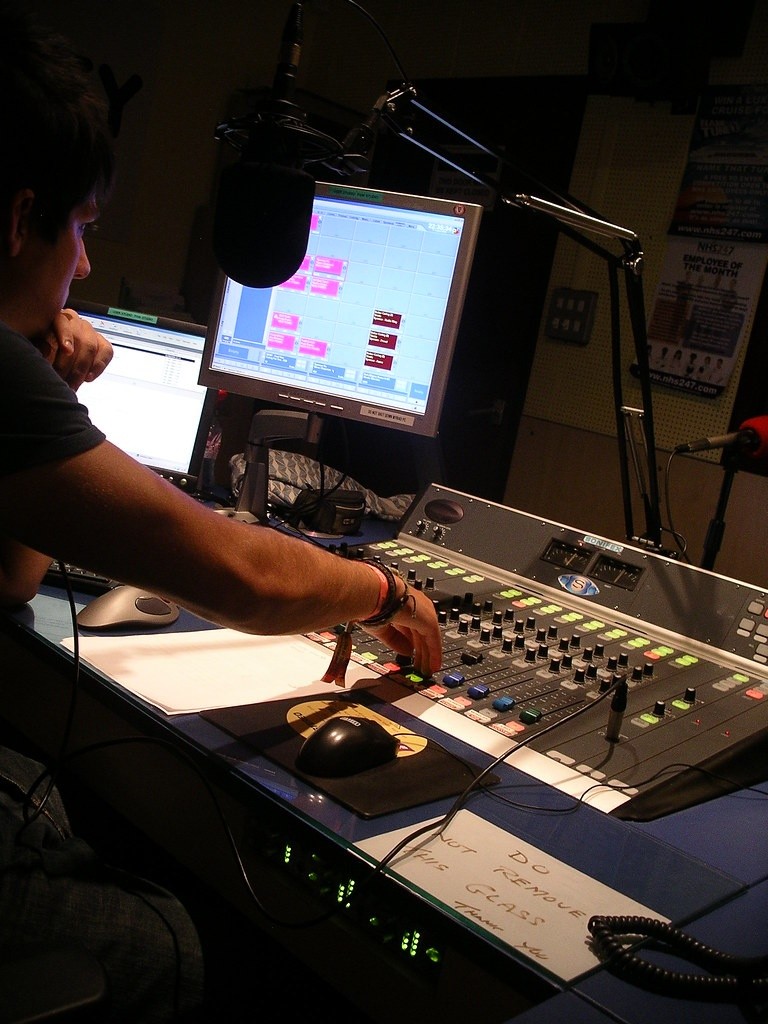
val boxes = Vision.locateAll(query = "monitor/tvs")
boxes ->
[197,182,483,439]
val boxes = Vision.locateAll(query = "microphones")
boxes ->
[675,415,768,459]
[212,5,315,287]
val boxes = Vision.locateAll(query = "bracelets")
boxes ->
[322,559,417,687]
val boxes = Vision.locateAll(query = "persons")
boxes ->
[0,38,443,1024]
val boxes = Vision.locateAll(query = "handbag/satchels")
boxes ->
[288,489,367,536]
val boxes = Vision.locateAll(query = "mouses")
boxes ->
[74,581,179,629]
[295,716,400,780]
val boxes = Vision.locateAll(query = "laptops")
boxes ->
[41,298,219,594]
